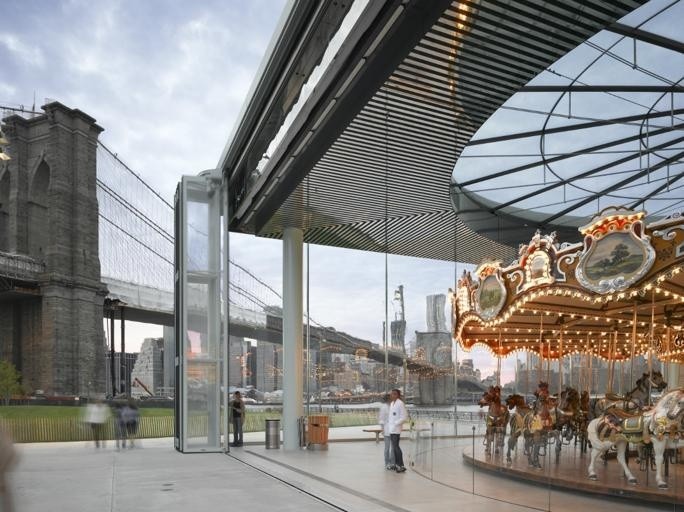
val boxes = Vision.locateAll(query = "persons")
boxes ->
[378,393,396,471]
[386,389,408,474]
[0,425,18,512]
[221,391,246,447]
[84,395,140,450]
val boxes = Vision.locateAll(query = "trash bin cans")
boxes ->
[264,418,281,449]
[307,413,329,446]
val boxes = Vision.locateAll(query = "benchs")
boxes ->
[362,427,430,445]
